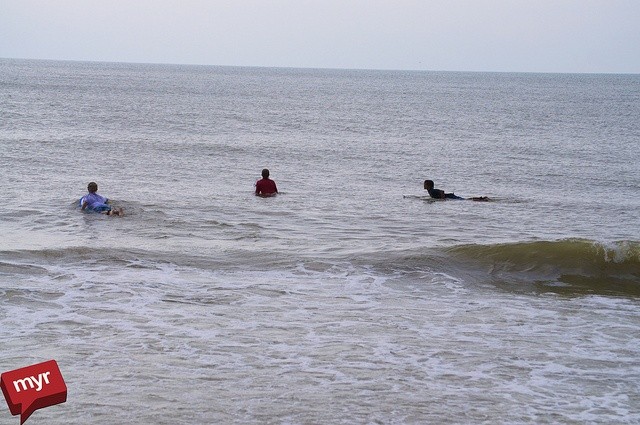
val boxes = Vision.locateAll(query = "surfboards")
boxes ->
[403,194,449,198]
[80,194,91,210]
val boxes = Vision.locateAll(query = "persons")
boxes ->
[255,169,280,196]
[423,180,487,201]
[82,182,125,216]
